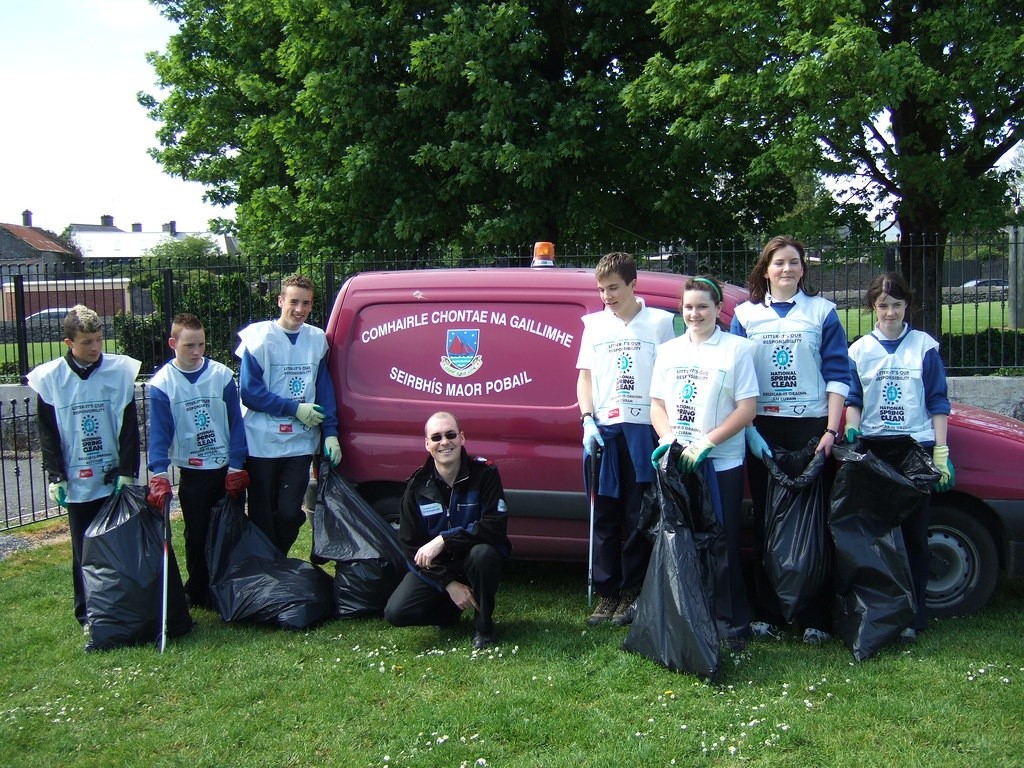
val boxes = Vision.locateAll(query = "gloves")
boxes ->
[746,426,773,460]
[932,445,955,493]
[840,425,862,444]
[294,402,327,428]
[223,470,250,500]
[651,435,676,472]
[677,434,717,474]
[49,480,68,509]
[583,420,604,455]
[114,475,136,498]
[146,477,174,514]
[323,435,342,468]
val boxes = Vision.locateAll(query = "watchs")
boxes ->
[824,428,839,439]
[580,412,594,424]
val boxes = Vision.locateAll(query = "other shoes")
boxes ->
[749,622,779,638]
[804,629,829,646]
[713,618,748,639]
[184,589,209,609]
[901,627,917,640]
[473,617,495,649]
[82,623,90,638]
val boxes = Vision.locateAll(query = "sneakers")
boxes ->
[611,588,643,624]
[586,590,622,624]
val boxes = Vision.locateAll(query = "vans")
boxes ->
[26,308,73,324]
[325,241,1024,627]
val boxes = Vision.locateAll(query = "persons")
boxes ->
[649,273,760,595]
[730,234,852,646]
[844,271,951,639]
[26,304,143,637]
[148,311,249,611]
[576,252,675,630]
[233,271,342,556]
[383,411,514,650]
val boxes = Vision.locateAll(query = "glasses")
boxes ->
[426,433,460,442]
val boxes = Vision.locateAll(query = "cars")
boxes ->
[958,279,1010,288]
